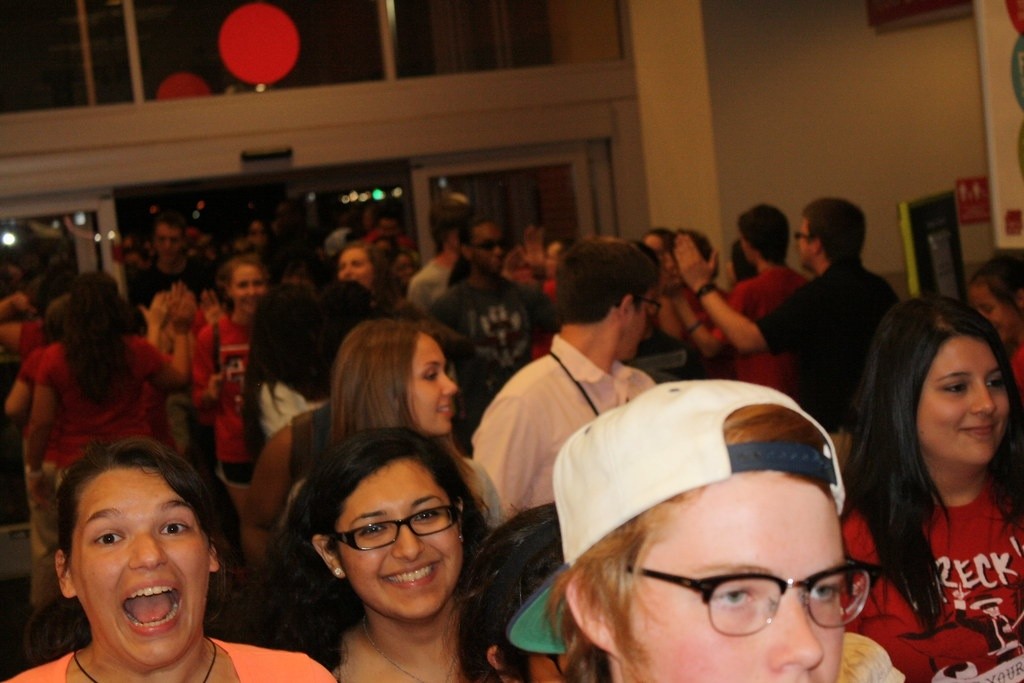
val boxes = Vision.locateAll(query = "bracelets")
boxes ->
[697,284,714,297]
[688,320,702,336]
[25,464,44,479]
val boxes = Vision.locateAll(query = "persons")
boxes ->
[0,435,337,683]
[967,255,1024,384]
[264,427,489,683]
[669,203,810,401]
[506,380,845,683]
[456,500,568,683]
[673,198,899,432]
[840,294,1024,683]
[277,318,486,529]
[0,204,758,606]
[469,236,662,518]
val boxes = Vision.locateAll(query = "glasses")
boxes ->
[325,501,459,552]
[794,231,807,240]
[468,237,505,253]
[620,556,879,638]
[630,290,661,321]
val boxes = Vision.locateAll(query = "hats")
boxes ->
[504,378,847,653]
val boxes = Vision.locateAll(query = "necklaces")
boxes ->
[362,622,459,683]
[549,352,599,417]
[72,636,217,683]
